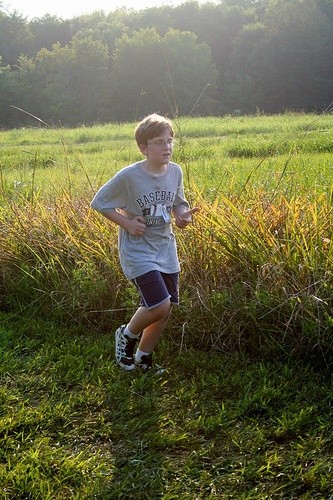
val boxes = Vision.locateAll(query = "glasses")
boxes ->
[146,138,174,149]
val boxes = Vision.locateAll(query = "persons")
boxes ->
[90,114,199,376]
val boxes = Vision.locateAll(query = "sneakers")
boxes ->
[115,324,139,371]
[133,352,165,375]
